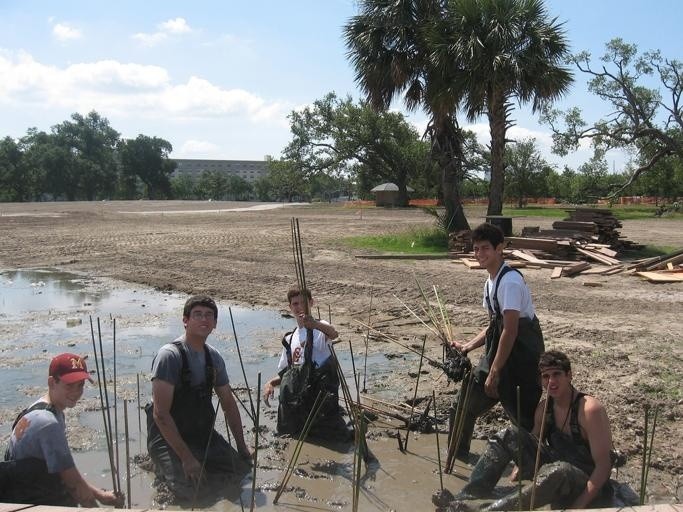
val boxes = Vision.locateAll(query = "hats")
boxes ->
[48,352,95,385]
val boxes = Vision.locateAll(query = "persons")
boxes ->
[4,353,124,508]
[261,286,361,440]
[449,222,545,460]
[141,296,250,499]
[432,348,611,510]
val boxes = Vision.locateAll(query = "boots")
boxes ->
[447,463,565,512]
[459,439,512,500]
